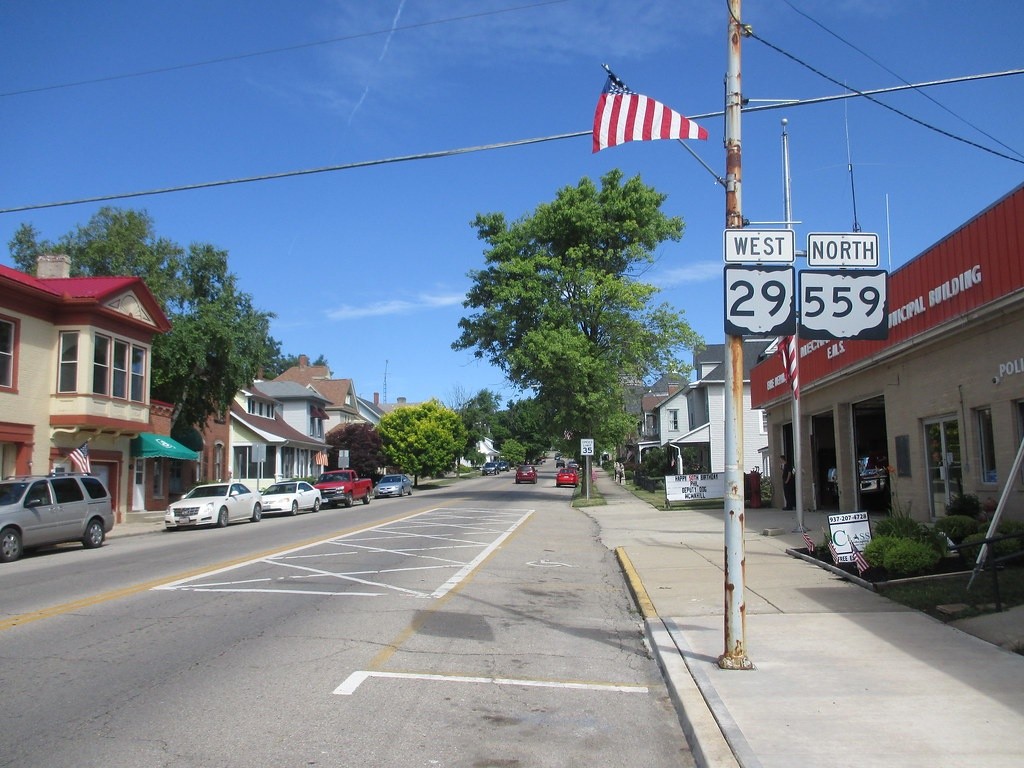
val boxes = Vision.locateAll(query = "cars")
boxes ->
[374,474,412,498]
[556,468,579,487]
[260,480,323,518]
[555,459,565,468]
[497,462,510,472]
[568,461,579,471]
[555,456,560,460]
[165,481,264,532]
[516,466,538,484]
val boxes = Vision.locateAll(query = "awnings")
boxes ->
[129,433,199,461]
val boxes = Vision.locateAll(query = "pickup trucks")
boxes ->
[313,469,372,510]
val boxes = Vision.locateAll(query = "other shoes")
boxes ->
[783,506,793,511]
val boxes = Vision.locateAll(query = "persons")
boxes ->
[779,455,794,511]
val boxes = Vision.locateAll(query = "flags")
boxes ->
[799,525,816,553]
[828,539,840,565]
[316,448,329,466]
[591,70,710,155]
[69,441,91,473]
[851,542,869,574]
[778,335,799,400]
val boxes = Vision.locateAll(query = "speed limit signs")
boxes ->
[580,439,594,455]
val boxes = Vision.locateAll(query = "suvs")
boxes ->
[482,462,499,476]
[0,471,116,563]
